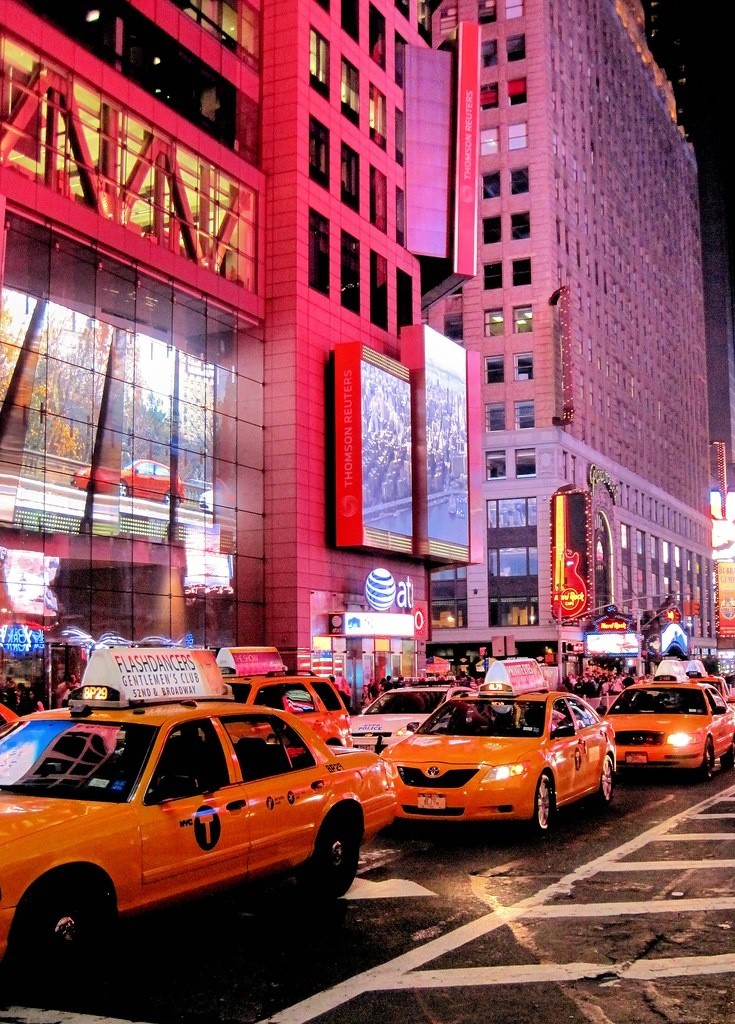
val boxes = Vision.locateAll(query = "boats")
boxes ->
[0,646,397,998]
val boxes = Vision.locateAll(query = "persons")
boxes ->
[64,736,135,791]
[57,674,80,707]
[563,663,656,716]
[382,696,410,713]
[520,709,545,734]
[487,712,514,736]
[128,744,190,797]
[362,670,457,709]
[458,670,483,692]
[725,675,735,687]
[196,719,242,785]
[552,701,564,712]
[0,677,46,716]
[330,676,339,693]
[285,669,298,676]
[265,671,276,677]
[179,727,207,767]
[268,734,276,743]
[708,666,714,676]
[460,693,470,698]
[437,709,475,736]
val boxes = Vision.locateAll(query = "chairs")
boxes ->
[68,731,282,791]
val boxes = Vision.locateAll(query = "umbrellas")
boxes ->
[427,656,448,663]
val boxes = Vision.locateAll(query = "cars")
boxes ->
[684,658,735,712]
[602,659,735,785]
[69,459,189,511]
[379,659,618,846]
[214,645,354,758]
[349,674,477,756]
